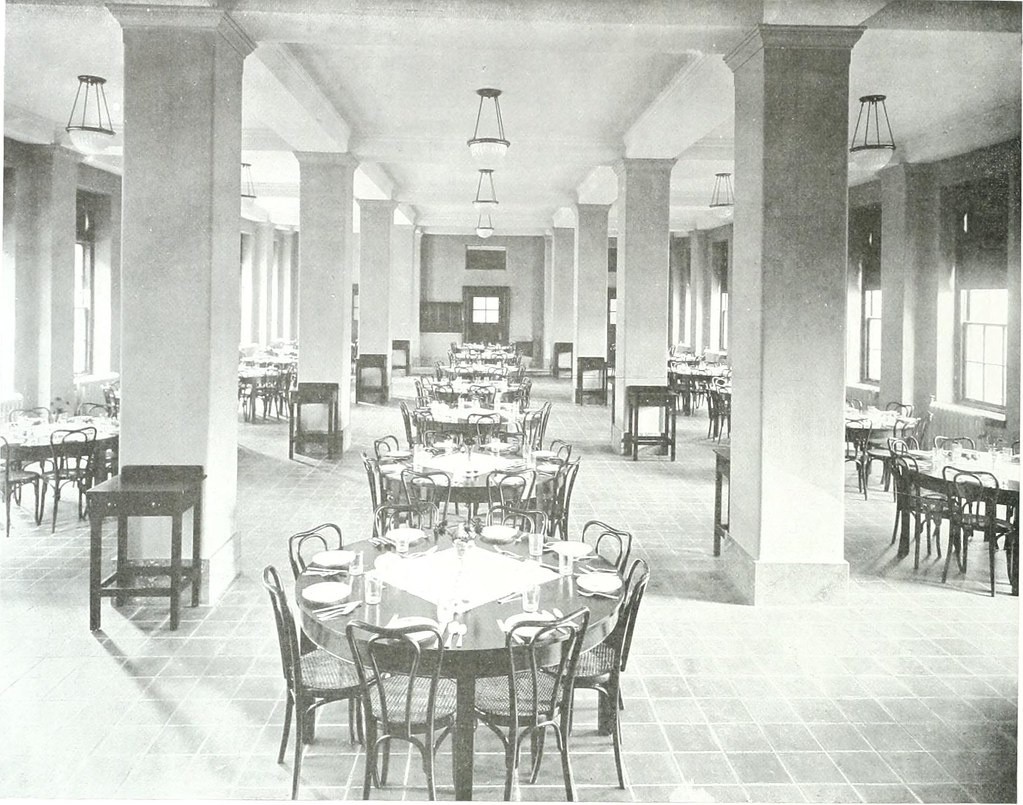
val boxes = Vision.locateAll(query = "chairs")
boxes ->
[465,607,590,801]
[531,560,651,789]
[343,618,459,801]
[0,324,1019,596]
[261,565,382,800]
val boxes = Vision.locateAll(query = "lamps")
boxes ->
[475,209,495,241]
[238,157,257,214]
[64,72,116,157]
[466,89,509,167]
[849,93,896,174]
[708,171,736,221]
[471,167,501,216]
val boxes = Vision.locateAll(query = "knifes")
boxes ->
[542,610,568,635]
[379,536,395,546]
[368,614,399,643]
[307,566,347,573]
[496,619,525,645]
[312,600,363,613]
[573,573,619,578]
[526,560,559,569]
[435,620,448,648]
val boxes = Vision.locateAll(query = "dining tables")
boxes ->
[290,528,625,800]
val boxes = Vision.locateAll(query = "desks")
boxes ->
[83,464,207,633]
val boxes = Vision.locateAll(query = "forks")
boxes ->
[573,555,598,561]
[493,544,522,558]
[577,590,619,599]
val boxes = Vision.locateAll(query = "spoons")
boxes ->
[553,608,578,628]
[457,624,467,648]
[515,532,527,542]
[579,567,617,575]
[444,621,459,649]
[302,570,339,577]
[586,564,618,572]
[369,537,387,547]
[317,603,359,620]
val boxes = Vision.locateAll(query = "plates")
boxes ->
[385,451,411,457]
[312,550,356,567]
[533,450,557,460]
[434,441,457,450]
[487,443,512,451]
[903,458,929,467]
[480,525,517,540]
[551,542,592,558]
[302,581,352,604]
[908,449,931,459]
[388,615,439,642]
[537,464,564,473]
[504,611,549,637]
[386,528,425,544]
[576,573,622,594]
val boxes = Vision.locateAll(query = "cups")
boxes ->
[526,452,537,470]
[1003,447,1012,461]
[491,438,500,454]
[396,535,409,558]
[445,439,453,456]
[349,550,364,576]
[365,569,383,605]
[522,584,541,612]
[559,550,574,576]
[529,534,544,556]
